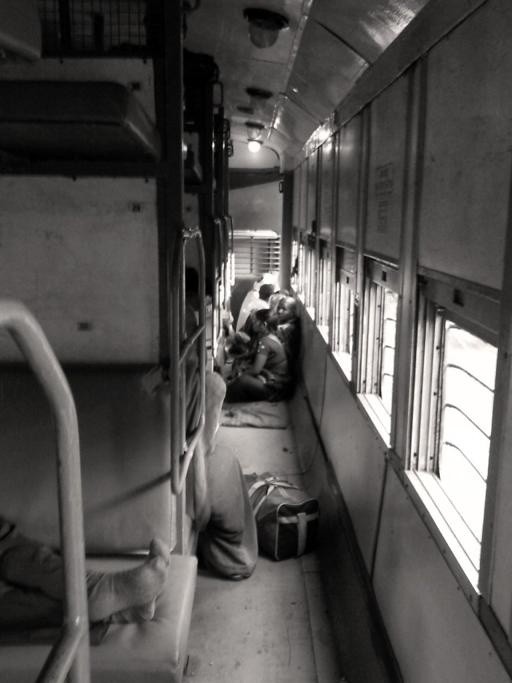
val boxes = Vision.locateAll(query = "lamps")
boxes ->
[235,7,291,155]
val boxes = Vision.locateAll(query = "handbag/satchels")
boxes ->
[243,472,319,562]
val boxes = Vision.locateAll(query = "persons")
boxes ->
[1,516,171,636]
[220,272,303,404]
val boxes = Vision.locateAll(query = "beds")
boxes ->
[0,550,199,682]
